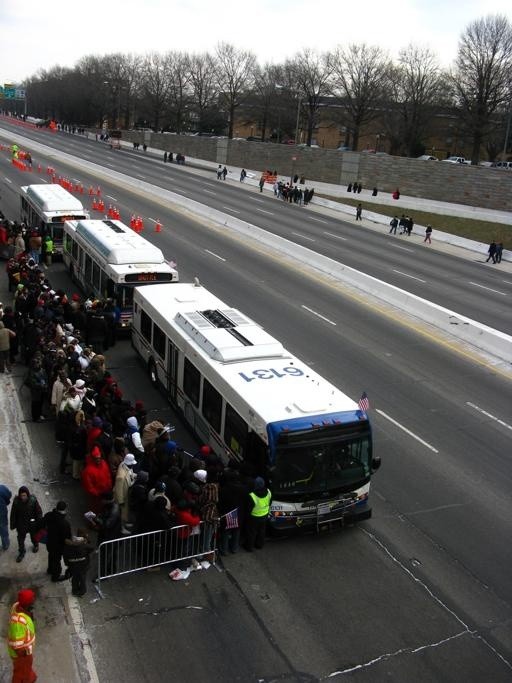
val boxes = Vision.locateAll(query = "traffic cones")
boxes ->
[58,175,83,192]
[89,184,160,233]
[0,143,56,183]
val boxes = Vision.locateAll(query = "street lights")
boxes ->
[295,89,306,143]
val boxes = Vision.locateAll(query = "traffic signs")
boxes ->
[0,83,25,102]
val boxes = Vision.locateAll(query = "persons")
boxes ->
[0,213,273,597]
[240,169,246,182]
[164,151,185,166]
[348,181,362,193]
[259,168,315,205]
[372,187,377,196]
[486,239,504,264]
[425,224,432,243]
[12,143,33,163]
[216,165,227,180]
[101,133,112,142]
[5,109,85,136]
[6,588,38,683]
[356,203,361,220]
[393,188,400,199]
[133,140,148,151]
[390,214,413,236]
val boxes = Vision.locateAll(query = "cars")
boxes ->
[138,128,215,136]
[283,138,391,156]
[416,152,512,173]
[211,135,262,142]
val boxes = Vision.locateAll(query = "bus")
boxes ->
[18,182,87,258]
[132,276,382,539]
[61,218,178,329]
